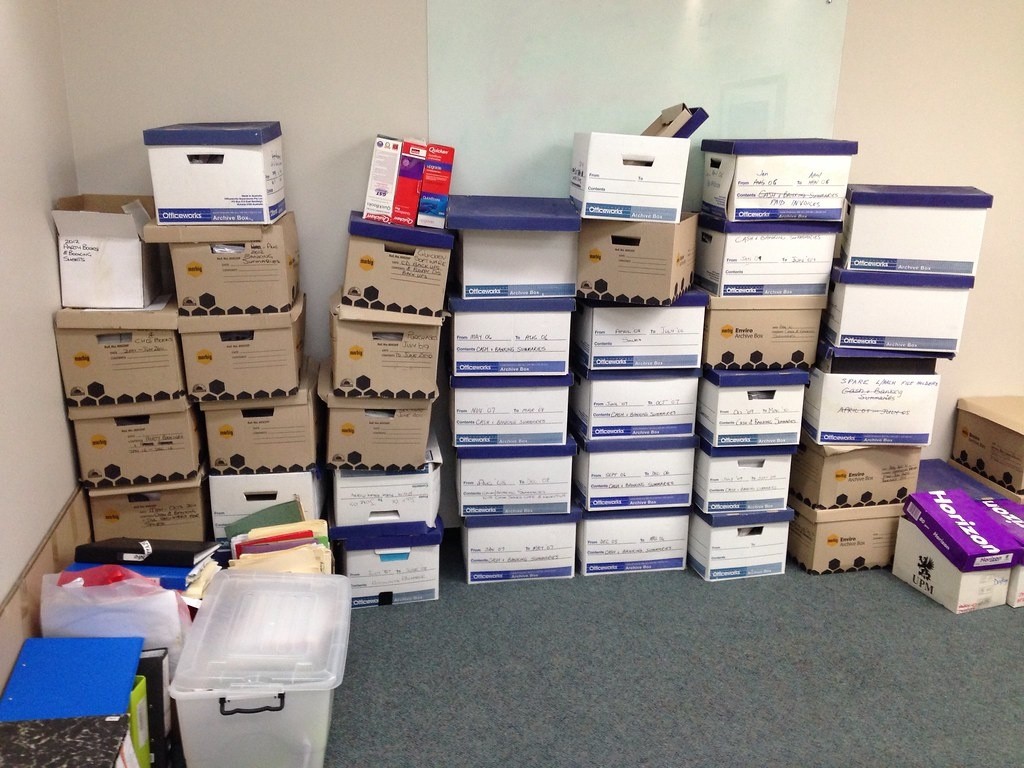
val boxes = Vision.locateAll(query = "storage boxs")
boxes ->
[693,214,844,298]
[701,289,827,371]
[462,506,584,585]
[819,185,993,352]
[50,121,456,610]
[572,433,701,511]
[688,370,808,582]
[801,337,955,446]
[447,292,577,375]
[446,194,581,300]
[892,396,1024,614]
[570,103,709,307]
[449,372,574,447]
[455,438,578,516]
[572,287,708,370]
[169,571,352,768]
[572,362,703,440]
[577,506,693,576]
[699,138,858,222]
[788,428,921,576]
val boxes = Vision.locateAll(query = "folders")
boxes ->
[62,536,224,591]
[128,647,174,768]
